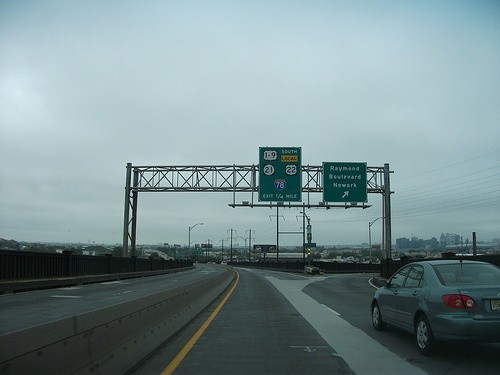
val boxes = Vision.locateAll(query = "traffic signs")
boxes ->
[322,161,367,203]
[259,146,302,201]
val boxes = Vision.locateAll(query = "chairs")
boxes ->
[475,273,496,283]
[440,273,457,283]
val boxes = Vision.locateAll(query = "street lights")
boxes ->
[369,216,385,265]
[299,211,312,266]
[188,222,203,259]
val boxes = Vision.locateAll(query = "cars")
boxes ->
[371,260,500,356]
[220,261,227,264]
